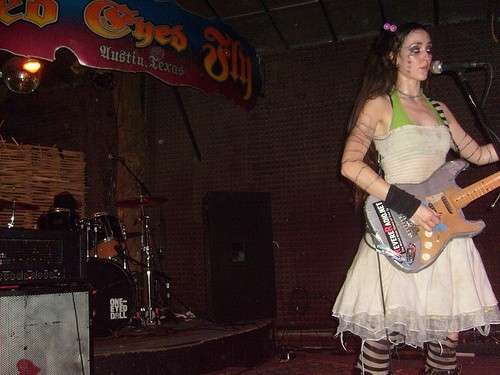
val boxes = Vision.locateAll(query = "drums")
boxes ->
[36,207,78,231]
[83,212,126,258]
[85,259,136,338]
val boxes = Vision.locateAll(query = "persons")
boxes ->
[331,17,500,375]
[33,191,84,231]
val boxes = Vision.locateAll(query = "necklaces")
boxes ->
[394,82,425,100]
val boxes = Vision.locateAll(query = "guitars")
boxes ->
[365,160,499,274]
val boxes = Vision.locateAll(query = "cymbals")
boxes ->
[0,200,39,211]
[113,196,168,207]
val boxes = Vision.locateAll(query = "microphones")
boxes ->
[430,60,489,74]
[108,153,124,162]
